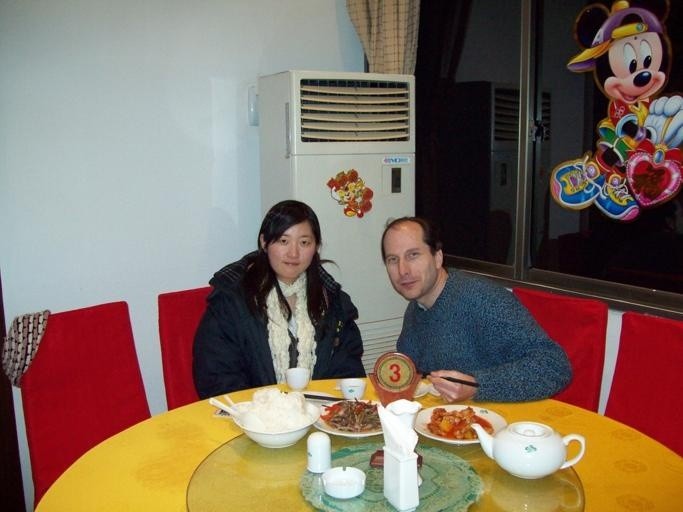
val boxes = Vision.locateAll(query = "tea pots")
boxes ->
[470,422,586,480]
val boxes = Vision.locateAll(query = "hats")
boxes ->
[566,1,664,73]
[0,310,53,387]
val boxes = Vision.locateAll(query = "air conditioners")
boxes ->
[258,70,415,375]
[439,78,550,259]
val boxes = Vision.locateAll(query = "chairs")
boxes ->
[21,301,151,511]
[158,286,214,411]
[603,311,682,459]
[512,287,608,413]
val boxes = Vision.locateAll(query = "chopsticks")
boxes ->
[418,370,479,387]
[304,392,342,401]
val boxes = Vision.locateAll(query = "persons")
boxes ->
[191,199,366,400]
[380,217,571,404]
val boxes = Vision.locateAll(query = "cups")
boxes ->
[284,367,311,390]
[340,378,366,399]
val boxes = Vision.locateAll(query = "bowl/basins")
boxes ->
[229,401,319,447]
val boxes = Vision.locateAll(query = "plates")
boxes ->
[413,403,506,445]
[312,399,382,437]
[412,381,428,399]
